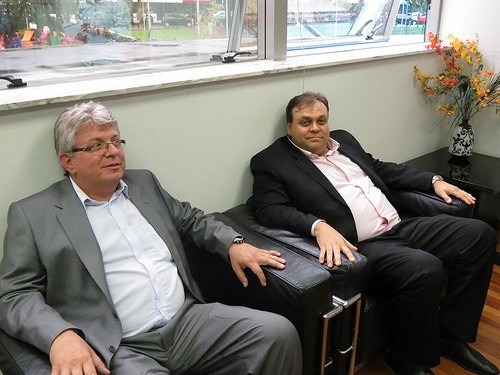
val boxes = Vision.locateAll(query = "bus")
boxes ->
[396,1,412,25]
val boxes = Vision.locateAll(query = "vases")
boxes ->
[447,118,475,157]
[443,156,472,181]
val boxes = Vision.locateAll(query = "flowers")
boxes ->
[413,29,500,136]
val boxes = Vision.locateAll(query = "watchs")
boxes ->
[430,175,444,185]
[229,236,247,244]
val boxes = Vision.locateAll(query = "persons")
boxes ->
[246,92,500,375]
[0,100,304,375]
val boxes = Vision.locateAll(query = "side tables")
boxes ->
[398,147,500,245]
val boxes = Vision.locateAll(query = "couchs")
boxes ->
[221,187,474,375]
[0,212,337,375]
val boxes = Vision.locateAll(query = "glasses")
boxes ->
[73,139,125,155]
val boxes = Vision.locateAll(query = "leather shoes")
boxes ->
[385,348,434,375]
[438,338,500,375]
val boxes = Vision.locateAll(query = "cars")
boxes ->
[410,12,428,26]
[211,10,234,27]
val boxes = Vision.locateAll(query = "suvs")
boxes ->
[162,12,194,28]
